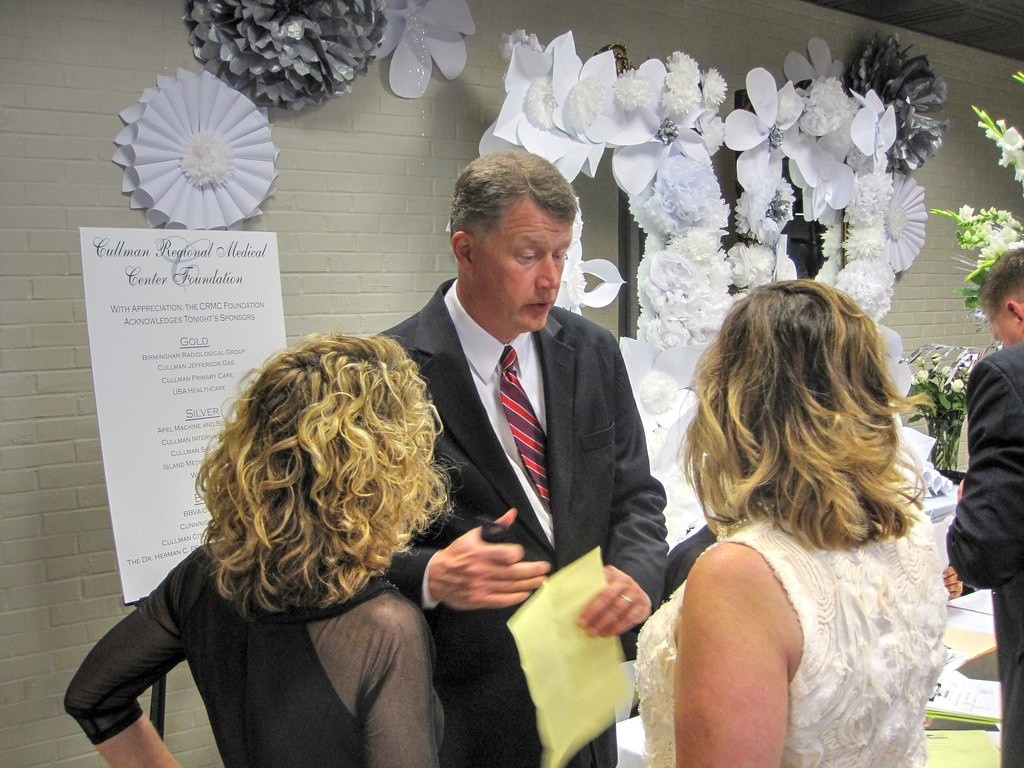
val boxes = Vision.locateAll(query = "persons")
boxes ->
[374,153,670,768]
[664,521,718,597]
[634,280,951,768]
[63,332,446,768]
[942,247,1024,768]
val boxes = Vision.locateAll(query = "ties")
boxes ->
[499,345,551,513]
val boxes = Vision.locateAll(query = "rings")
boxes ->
[620,594,632,603]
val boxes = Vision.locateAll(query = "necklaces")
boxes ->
[727,495,792,534]
